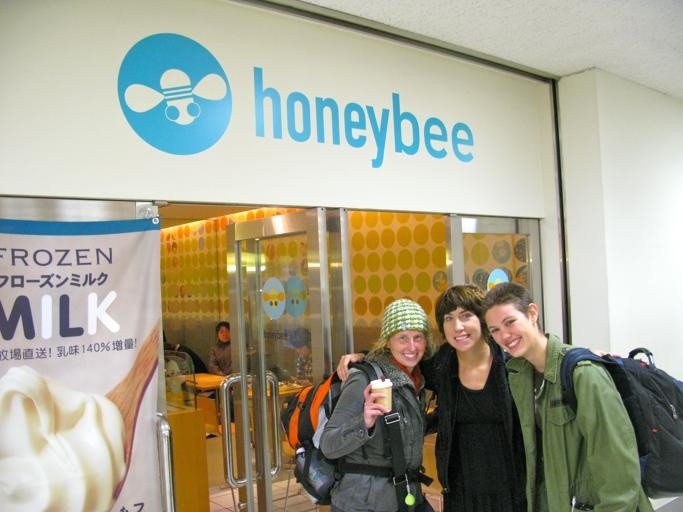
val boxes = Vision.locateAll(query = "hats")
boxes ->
[379,298,429,338]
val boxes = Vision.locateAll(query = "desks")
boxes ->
[164,371,307,400]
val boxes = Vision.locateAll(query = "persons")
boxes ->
[207,322,252,438]
[165,342,207,395]
[319,298,440,512]
[483,281,655,512]
[283,326,334,386]
[336,285,527,512]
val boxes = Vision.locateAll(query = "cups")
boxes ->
[370,378,393,415]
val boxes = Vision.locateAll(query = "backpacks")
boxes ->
[560,346,683,498]
[280,357,412,507]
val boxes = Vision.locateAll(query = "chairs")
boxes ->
[179,393,254,436]
[266,403,300,512]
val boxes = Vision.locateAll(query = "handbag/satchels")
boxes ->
[422,379,441,436]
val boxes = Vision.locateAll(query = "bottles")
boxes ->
[294,447,332,499]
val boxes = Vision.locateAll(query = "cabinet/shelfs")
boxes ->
[167,403,210,512]
